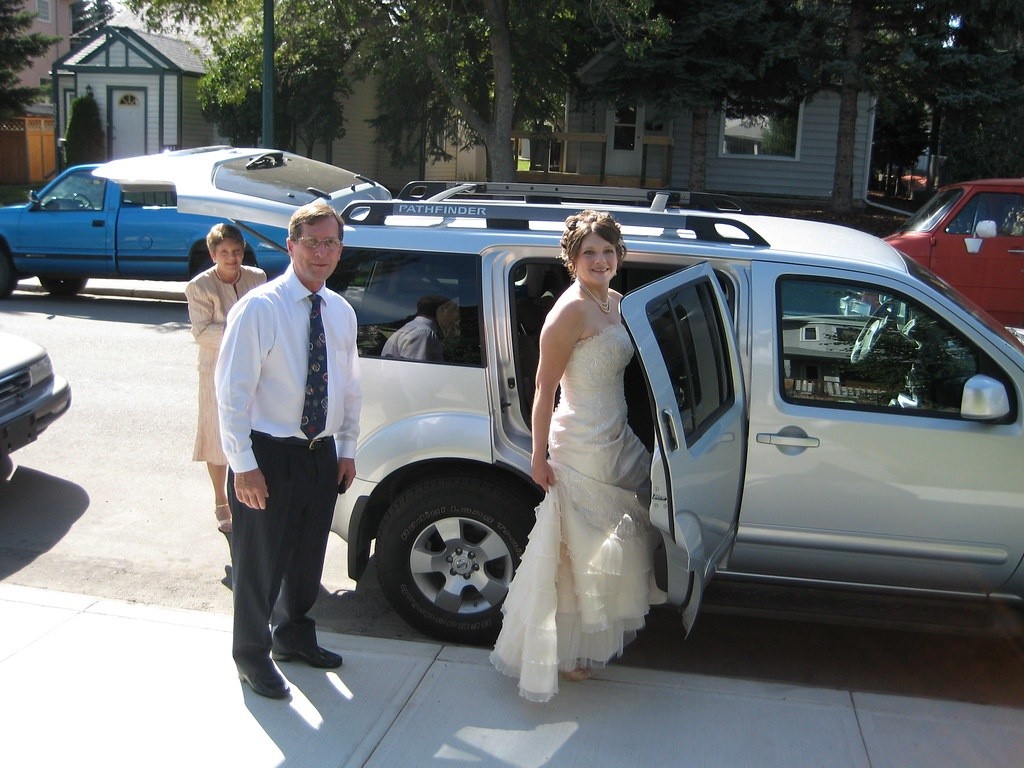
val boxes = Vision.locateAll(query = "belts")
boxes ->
[252,430,333,449]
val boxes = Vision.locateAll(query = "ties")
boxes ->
[300,293,328,442]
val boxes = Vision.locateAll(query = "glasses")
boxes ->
[297,238,340,248]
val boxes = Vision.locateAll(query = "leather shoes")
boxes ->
[273,646,343,669]
[239,666,290,696]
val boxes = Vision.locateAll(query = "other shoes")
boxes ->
[562,668,591,681]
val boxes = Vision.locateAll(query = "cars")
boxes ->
[0,331,71,456]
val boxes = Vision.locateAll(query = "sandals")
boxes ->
[215,503,233,532]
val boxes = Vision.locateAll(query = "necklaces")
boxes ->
[575,277,611,315]
[214,264,240,285]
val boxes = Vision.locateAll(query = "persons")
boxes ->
[376,295,456,363]
[214,197,365,700]
[183,220,268,534]
[487,209,669,704]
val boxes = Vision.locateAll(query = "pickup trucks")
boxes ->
[0,163,291,299]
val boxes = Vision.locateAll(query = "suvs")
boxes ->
[91,146,1024,645]
[841,178,1024,327]
